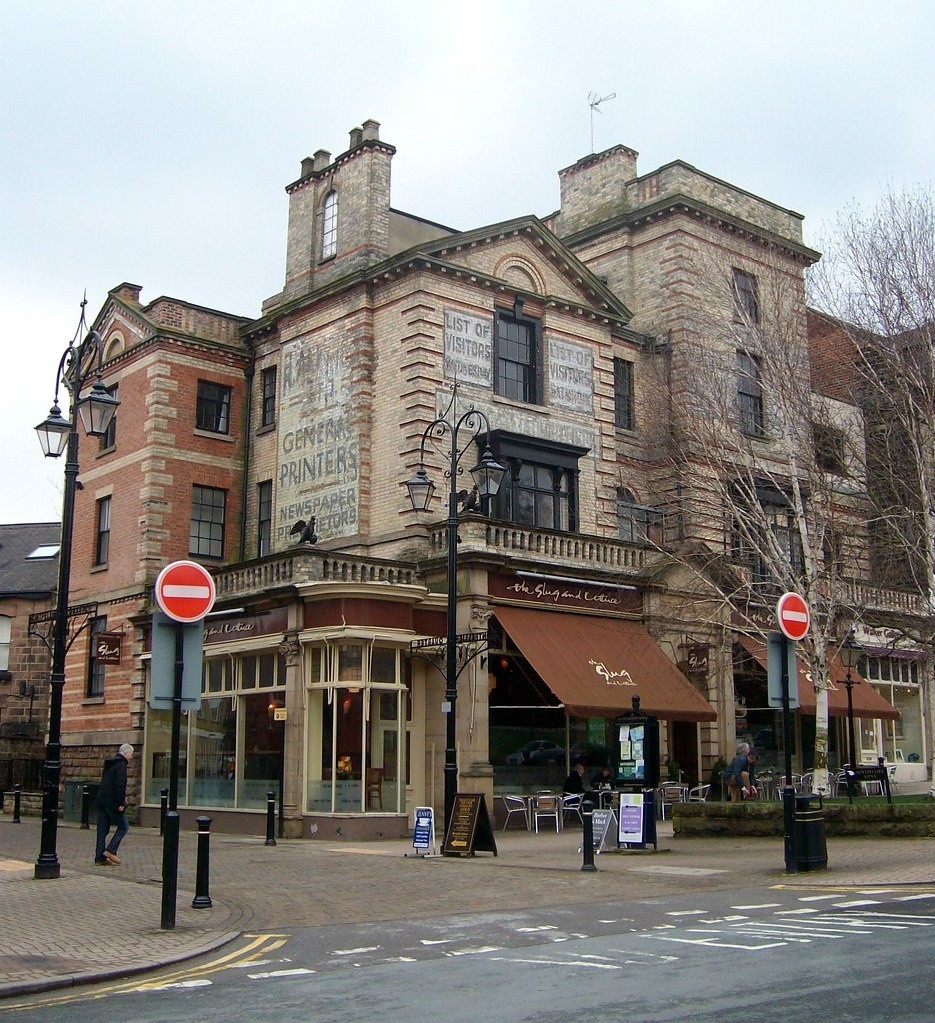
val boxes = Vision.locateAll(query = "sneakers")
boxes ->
[95,857,112,866]
[103,851,122,864]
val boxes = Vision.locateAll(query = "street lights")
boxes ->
[403,371,508,856]
[30,286,121,881]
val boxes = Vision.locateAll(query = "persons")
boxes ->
[724,743,760,803]
[563,764,616,813]
[95,743,134,865]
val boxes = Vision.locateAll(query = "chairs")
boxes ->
[366,767,383,811]
[503,781,711,834]
[755,763,899,800]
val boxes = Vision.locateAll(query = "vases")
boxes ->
[338,772,348,780]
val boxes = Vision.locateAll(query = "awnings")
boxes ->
[487,607,718,722]
[738,634,900,719]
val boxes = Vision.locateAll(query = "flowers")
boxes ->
[338,755,353,775]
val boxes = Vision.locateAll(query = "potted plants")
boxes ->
[709,755,729,801]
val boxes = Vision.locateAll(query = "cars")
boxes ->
[505,740,564,768]
[570,741,612,765]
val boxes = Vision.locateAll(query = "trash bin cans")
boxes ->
[794,793,828,871]
[63,780,100,823]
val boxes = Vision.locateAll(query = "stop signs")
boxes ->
[776,591,811,642]
[154,559,217,624]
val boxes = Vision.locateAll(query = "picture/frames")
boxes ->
[884,711,906,740]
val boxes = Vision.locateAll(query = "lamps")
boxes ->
[267,704,275,730]
[342,701,351,719]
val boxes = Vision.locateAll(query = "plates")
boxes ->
[417,824,429,826]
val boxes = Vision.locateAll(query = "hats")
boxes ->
[603,766,612,772]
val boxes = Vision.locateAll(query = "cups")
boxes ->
[419,818,430,824]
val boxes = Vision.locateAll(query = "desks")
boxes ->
[153,750,280,779]
[528,794,564,831]
[590,787,632,809]
